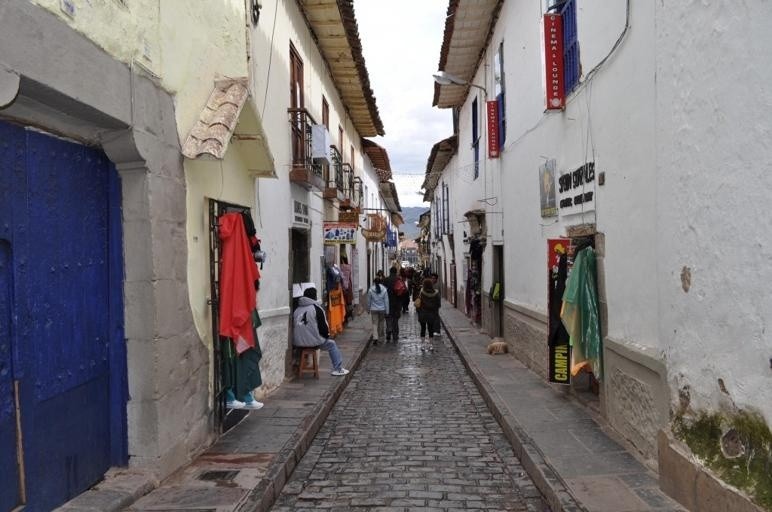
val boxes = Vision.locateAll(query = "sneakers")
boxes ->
[226,400,264,410]
[421,332,441,351]
[373,336,399,345]
[331,368,350,376]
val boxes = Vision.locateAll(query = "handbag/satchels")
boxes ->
[393,279,407,296]
[414,296,421,308]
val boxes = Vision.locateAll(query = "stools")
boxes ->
[300,350,319,379]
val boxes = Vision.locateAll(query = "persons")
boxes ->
[291,286,349,378]
[340,255,353,313]
[224,388,264,411]
[327,264,341,290]
[468,269,481,330]
[365,262,444,351]
